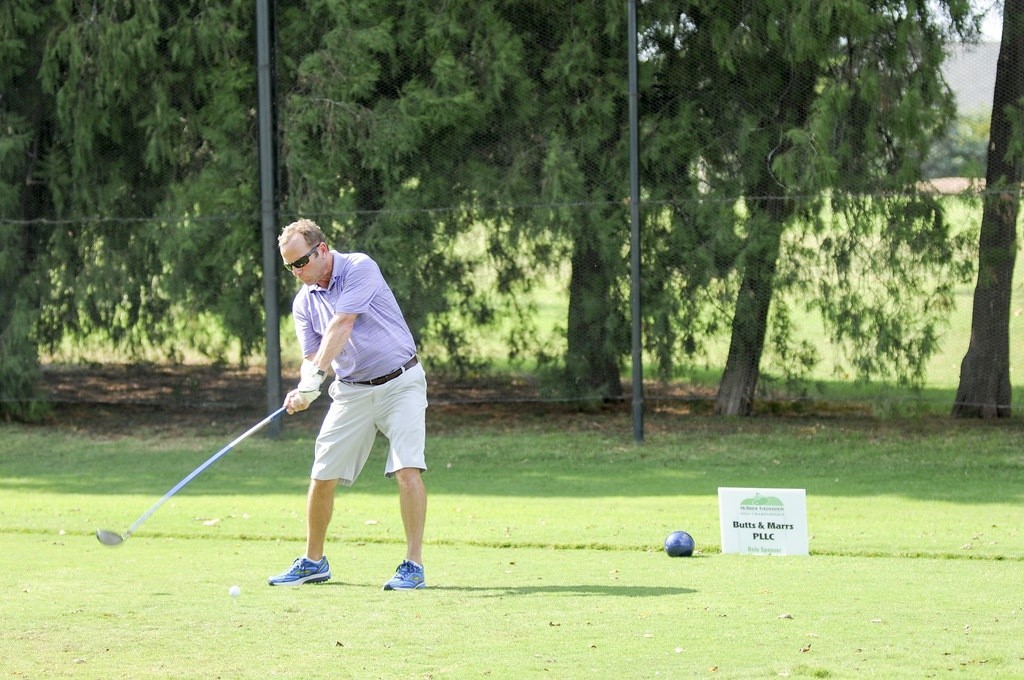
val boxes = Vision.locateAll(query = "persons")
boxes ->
[268,217,428,592]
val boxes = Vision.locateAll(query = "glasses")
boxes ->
[283,244,324,272]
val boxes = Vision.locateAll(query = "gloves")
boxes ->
[297,368,327,403]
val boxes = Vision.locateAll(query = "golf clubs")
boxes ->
[95,390,322,546]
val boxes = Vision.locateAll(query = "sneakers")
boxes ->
[382,560,426,591]
[268,556,331,586]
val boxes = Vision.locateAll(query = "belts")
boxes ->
[347,354,418,388]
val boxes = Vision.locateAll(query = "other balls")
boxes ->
[665,531,695,557]
